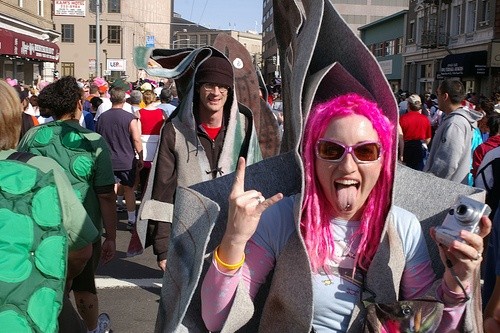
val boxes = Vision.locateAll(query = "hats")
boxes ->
[76,80,91,90]
[405,94,422,105]
[12,85,28,103]
[140,83,153,91]
[195,56,233,90]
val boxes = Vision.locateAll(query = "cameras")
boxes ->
[436,193,492,250]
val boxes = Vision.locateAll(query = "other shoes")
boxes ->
[115,202,128,211]
[127,221,135,230]
[135,191,143,203]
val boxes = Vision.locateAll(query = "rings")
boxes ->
[256,196,261,204]
[476,253,481,259]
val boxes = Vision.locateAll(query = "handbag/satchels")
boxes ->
[347,296,444,333]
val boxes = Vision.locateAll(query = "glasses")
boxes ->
[196,82,230,93]
[315,138,382,163]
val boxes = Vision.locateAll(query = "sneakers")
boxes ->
[94,313,110,333]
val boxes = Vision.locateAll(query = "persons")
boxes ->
[0,69,178,333]
[96,87,143,226]
[391,77,500,333]
[130,45,264,273]
[259,80,284,141]
[154,0,491,333]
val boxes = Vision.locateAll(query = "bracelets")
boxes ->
[212,244,246,269]
